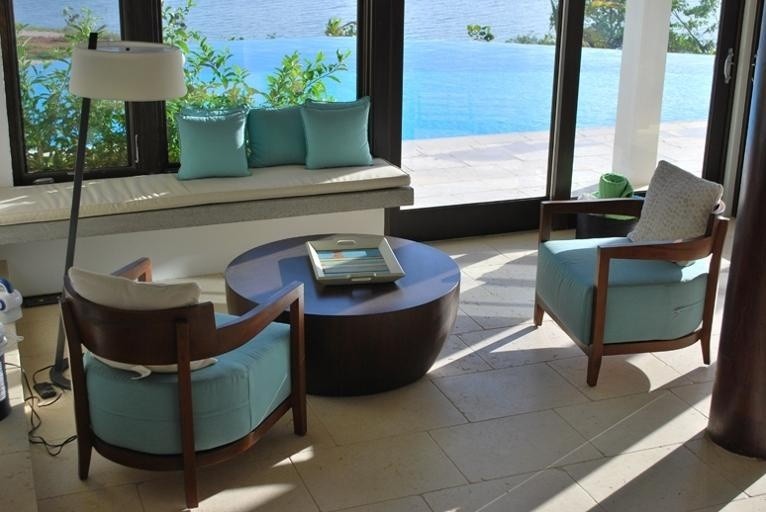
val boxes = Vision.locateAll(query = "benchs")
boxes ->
[0,158,417,247]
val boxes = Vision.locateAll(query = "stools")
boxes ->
[576,190,646,237]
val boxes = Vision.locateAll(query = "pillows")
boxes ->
[170,108,250,181]
[242,102,309,169]
[178,105,251,123]
[297,102,377,170]
[625,161,726,271]
[304,94,371,110]
[61,261,218,380]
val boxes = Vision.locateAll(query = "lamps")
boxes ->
[47,32,187,391]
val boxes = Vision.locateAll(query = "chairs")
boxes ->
[52,255,310,511]
[531,160,730,389]
